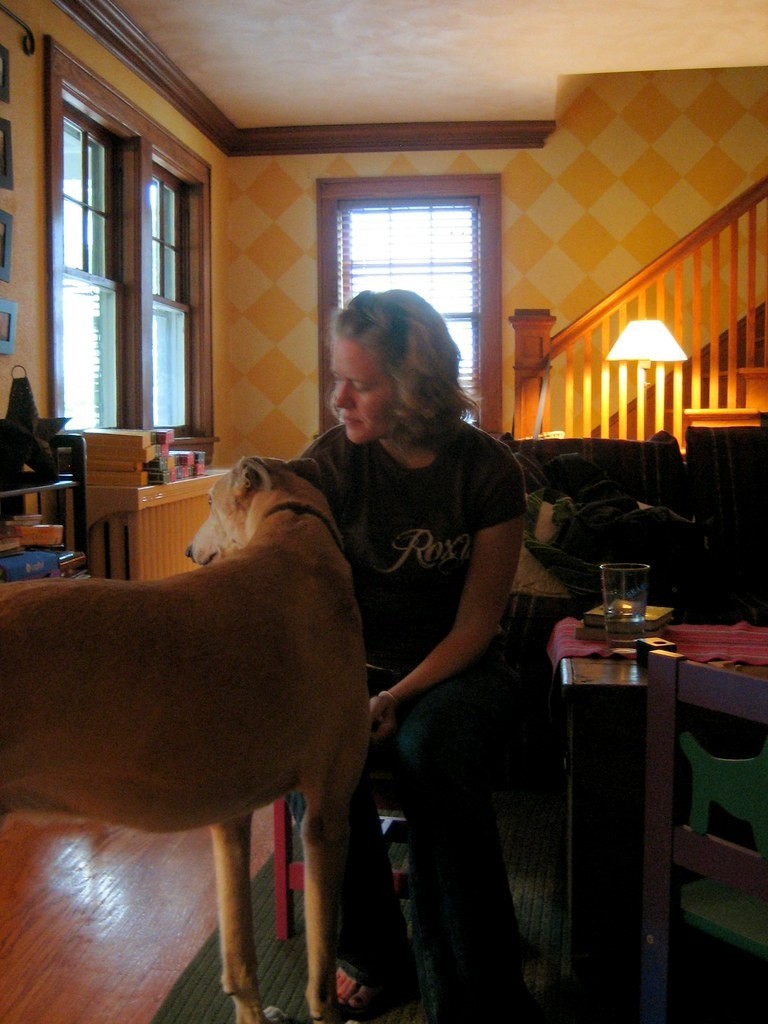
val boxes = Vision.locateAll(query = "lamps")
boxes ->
[604,315,689,439]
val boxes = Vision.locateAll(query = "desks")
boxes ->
[545,614,766,983]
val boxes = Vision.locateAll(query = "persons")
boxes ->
[289,291,548,1024]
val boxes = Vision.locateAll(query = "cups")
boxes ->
[599,563,650,647]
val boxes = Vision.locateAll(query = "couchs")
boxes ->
[478,418,767,787]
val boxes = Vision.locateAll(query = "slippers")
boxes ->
[333,968,415,1021]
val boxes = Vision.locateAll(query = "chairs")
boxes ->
[637,648,768,1022]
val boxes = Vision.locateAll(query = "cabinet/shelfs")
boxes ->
[0,430,91,584]
[89,488,224,583]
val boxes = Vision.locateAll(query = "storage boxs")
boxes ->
[83,425,206,488]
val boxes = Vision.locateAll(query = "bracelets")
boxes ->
[378,690,397,704]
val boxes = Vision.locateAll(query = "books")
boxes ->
[575,596,675,642]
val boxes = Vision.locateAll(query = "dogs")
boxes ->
[0,456,376,1024]
